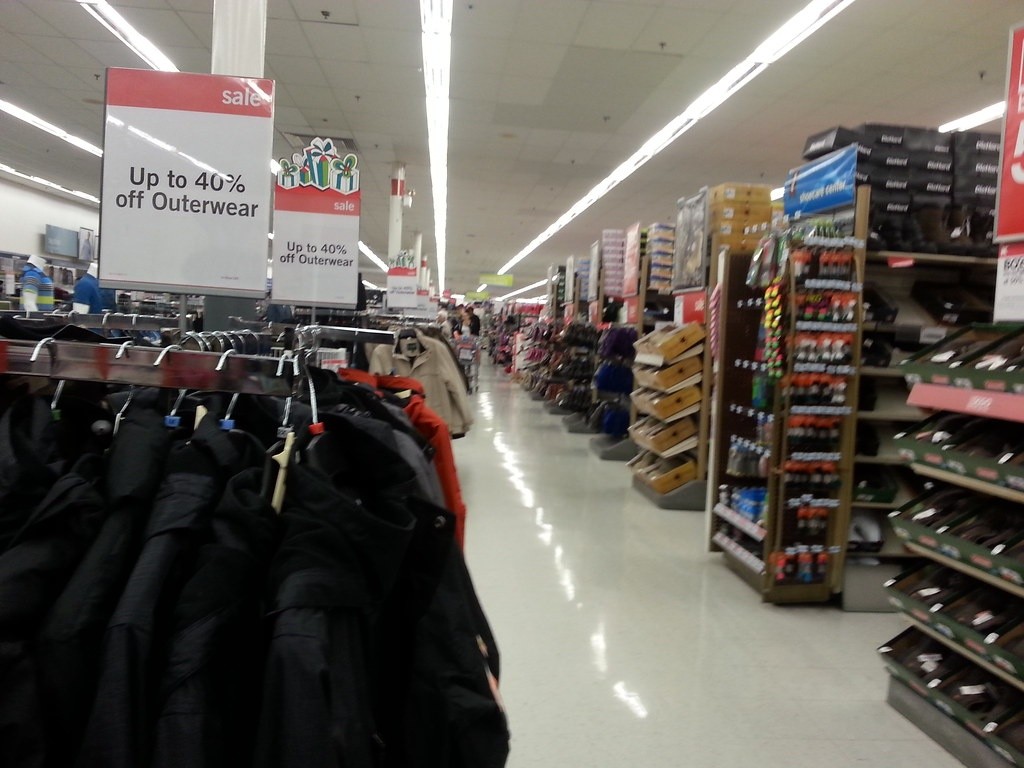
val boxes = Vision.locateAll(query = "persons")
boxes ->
[81,232,92,260]
[19,255,54,312]
[436,307,480,340]
[73,263,115,336]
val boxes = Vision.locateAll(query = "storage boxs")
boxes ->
[706,182,785,255]
[784,122,1000,215]
[878,326,1024,767]
[627,322,706,495]
[564,221,675,303]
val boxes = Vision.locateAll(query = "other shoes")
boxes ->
[450,335,455,338]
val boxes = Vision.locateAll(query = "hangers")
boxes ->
[1,302,449,511]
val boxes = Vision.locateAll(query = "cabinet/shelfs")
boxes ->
[885,382,1024,767]
[708,236,860,607]
[549,256,674,461]
[841,251,996,614]
[632,345,705,512]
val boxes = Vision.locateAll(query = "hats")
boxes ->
[465,306,473,312]
